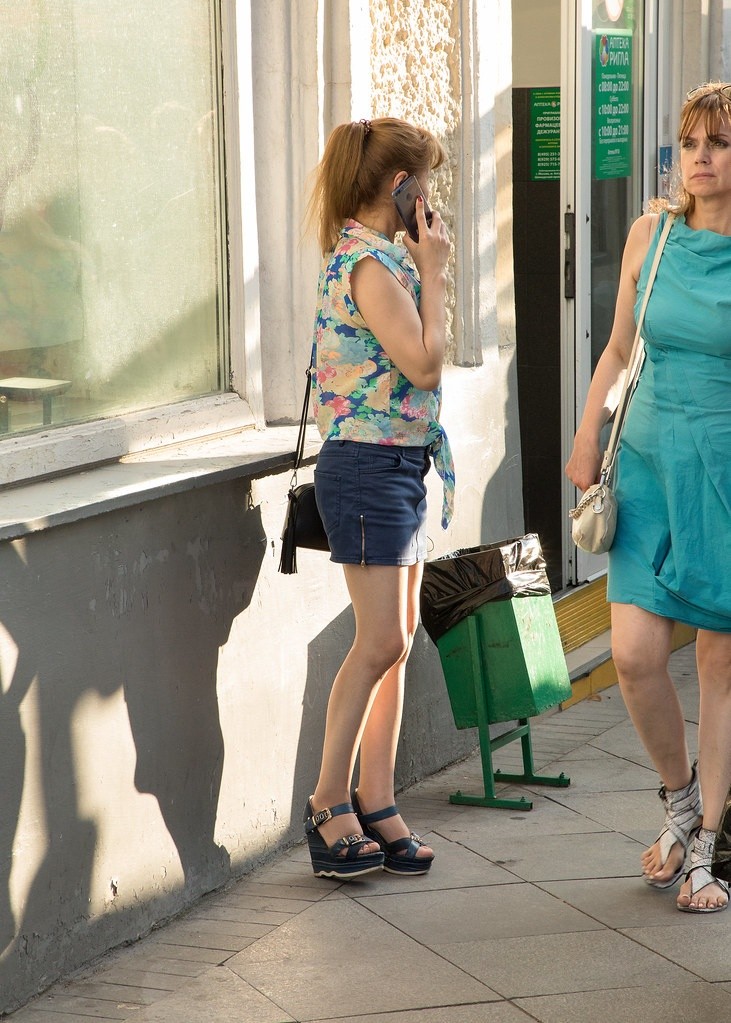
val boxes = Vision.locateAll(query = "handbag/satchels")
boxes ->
[569,481,619,556]
[278,482,332,574]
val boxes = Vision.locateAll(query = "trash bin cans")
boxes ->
[420,533,573,811]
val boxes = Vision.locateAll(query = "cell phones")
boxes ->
[391,174,433,243]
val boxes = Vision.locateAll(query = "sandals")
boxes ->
[676,827,728,912]
[641,761,703,889]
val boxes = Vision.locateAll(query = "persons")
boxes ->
[304,119,450,880]
[566,82,731,916]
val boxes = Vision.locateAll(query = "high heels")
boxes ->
[350,787,434,876]
[301,795,384,876]
